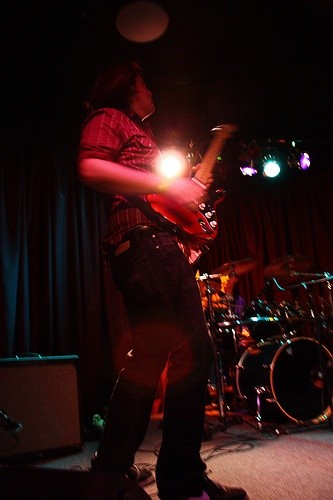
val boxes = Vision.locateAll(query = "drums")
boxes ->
[235,336,333,426]
[246,299,304,342]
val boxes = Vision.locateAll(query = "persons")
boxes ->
[77,62,247,500]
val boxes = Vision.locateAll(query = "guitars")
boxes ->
[144,121,235,244]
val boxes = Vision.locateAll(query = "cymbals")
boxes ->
[211,255,255,276]
[264,255,315,279]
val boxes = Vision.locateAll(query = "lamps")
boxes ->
[287,143,311,171]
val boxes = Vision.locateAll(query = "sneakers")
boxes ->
[188,474,247,500]
[133,466,155,487]
